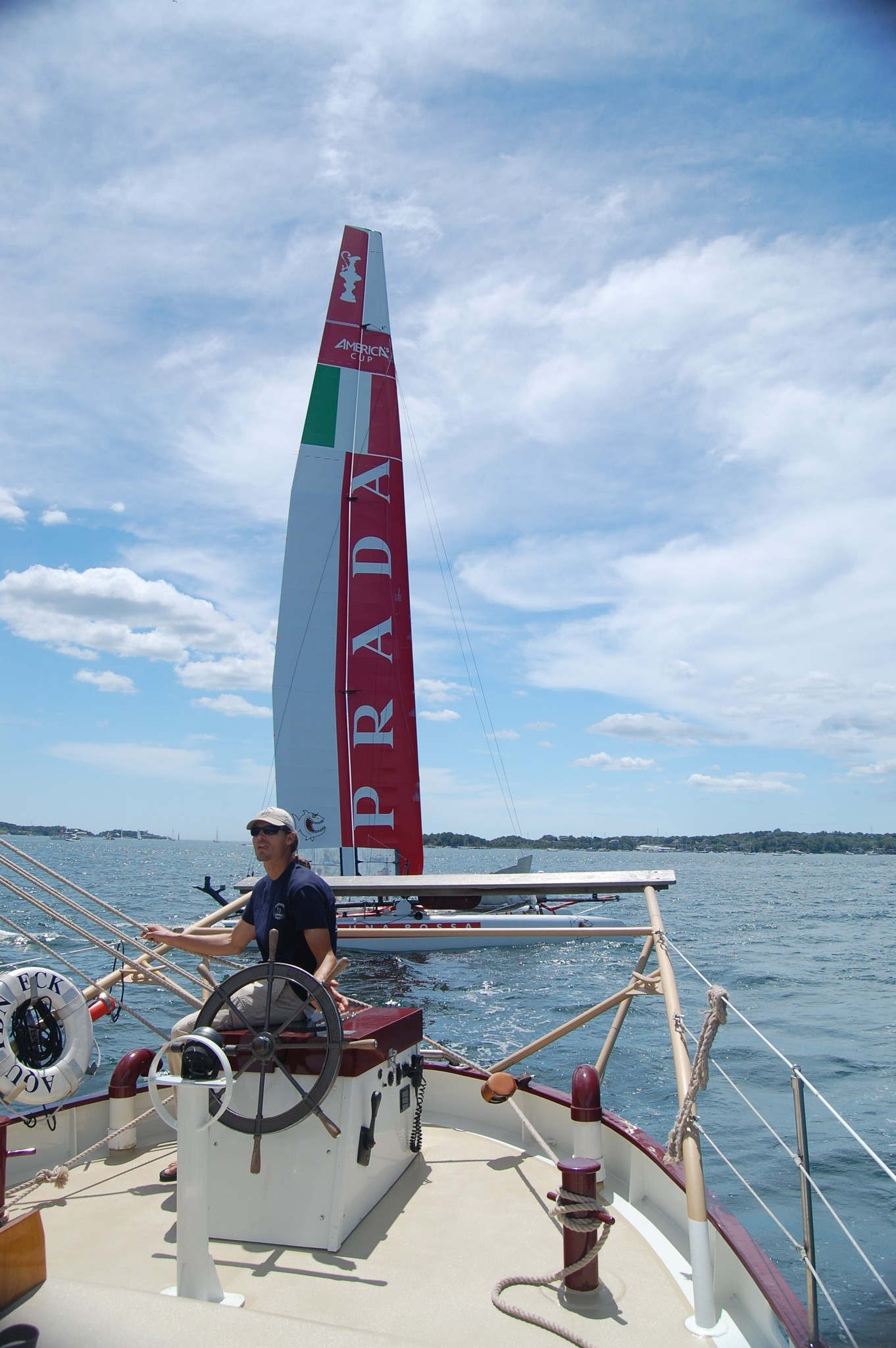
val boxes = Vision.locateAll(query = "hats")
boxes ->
[246,807,294,831]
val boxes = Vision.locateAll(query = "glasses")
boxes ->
[251,826,288,836]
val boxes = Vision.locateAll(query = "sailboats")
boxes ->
[190,220,641,955]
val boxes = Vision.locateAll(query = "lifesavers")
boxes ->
[0,966,97,1106]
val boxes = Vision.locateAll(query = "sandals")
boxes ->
[159,1167,177,1182]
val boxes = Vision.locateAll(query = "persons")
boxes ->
[140,807,349,1181]
